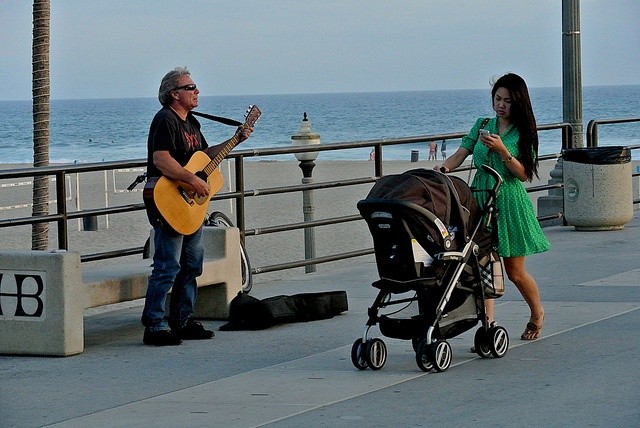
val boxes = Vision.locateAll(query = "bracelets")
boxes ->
[502,154,513,164]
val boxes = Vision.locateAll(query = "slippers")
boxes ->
[521,314,544,340]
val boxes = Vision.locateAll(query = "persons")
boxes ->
[441,140,448,161]
[142,67,254,346]
[433,73,550,353]
[370,149,375,161]
[428,141,435,161]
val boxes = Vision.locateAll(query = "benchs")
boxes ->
[0,225,243,356]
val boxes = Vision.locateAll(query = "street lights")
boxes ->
[291,110,322,273]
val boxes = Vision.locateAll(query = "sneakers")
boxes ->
[173,322,215,340]
[143,327,183,346]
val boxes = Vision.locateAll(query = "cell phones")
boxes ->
[478,128,492,143]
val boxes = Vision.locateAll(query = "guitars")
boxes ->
[143,105,261,237]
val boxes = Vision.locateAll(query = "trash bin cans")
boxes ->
[562,146,633,231]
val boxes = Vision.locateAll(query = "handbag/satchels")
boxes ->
[462,248,505,300]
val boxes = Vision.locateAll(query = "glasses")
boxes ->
[175,84,196,91]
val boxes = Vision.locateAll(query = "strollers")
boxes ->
[353,163,507,371]
